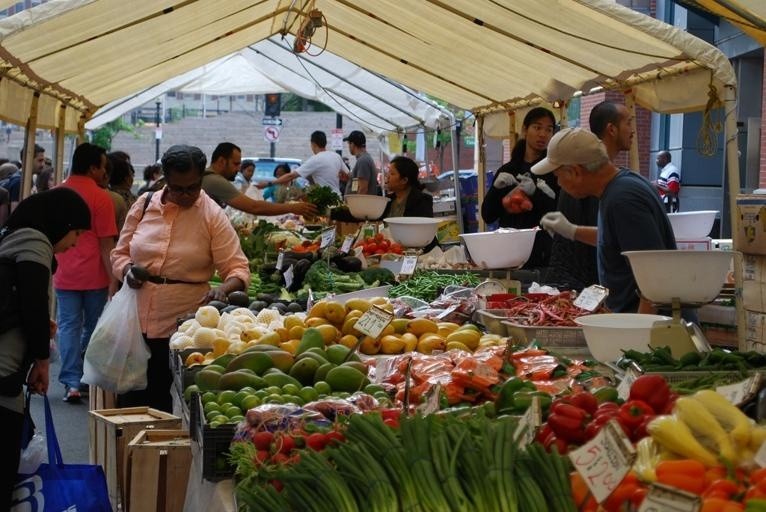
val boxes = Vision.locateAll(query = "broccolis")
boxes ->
[304,259,365,294]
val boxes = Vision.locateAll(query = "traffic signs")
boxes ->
[262,118,282,126]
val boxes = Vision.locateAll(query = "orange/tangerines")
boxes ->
[201,382,391,428]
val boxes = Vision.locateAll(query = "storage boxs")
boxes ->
[733,305,765,357]
[86,382,117,412]
[86,402,182,512]
[123,428,194,511]
[730,192,765,255]
[730,247,766,314]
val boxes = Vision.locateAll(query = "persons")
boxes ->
[0,129,439,403]
[104,143,253,418]
[0,185,93,512]
[544,100,636,294]
[649,149,681,214]
[479,107,563,270]
[529,125,701,329]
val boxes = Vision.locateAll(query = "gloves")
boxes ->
[493,172,517,189]
[540,211,577,242]
[516,173,536,195]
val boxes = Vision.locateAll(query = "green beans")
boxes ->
[387,267,483,300]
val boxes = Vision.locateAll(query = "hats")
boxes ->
[528,125,608,176]
[343,130,366,146]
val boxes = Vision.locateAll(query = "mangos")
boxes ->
[184,297,500,401]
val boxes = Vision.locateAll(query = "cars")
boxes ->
[436,167,482,185]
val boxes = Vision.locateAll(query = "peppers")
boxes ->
[475,344,766,512]
[503,290,613,326]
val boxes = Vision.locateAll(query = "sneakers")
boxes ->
[62,386,82,402]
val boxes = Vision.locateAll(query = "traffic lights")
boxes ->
[264,91,281,116]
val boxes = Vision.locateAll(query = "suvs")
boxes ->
[238,156,311,202]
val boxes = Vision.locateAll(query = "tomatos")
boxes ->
[357,233,402,256]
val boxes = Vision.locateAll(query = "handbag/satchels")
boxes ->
[10,459,114,512]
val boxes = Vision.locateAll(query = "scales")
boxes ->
[620,249,732,361]
[344,195,391,248]
[379,217,444,276]
[457,226,542,310]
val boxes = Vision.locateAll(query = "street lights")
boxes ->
[152,93,163,164]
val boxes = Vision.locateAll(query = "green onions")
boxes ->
[238,408,577,512]
